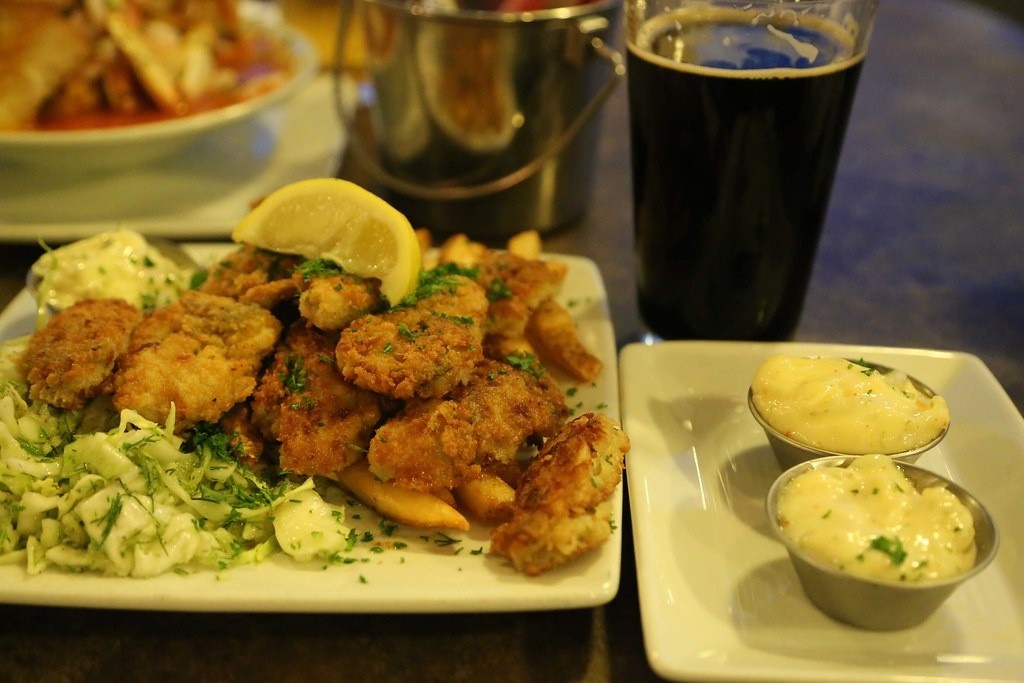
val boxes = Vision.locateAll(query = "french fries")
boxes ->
[336,456,520,530]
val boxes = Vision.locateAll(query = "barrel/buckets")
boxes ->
[332,0,625,247]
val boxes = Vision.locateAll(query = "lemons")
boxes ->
[230,177,422,308]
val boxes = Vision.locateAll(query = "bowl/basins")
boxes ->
[0,0,318,169]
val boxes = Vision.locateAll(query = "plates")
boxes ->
[0,72,344,242]
[619,341,1024,683]
[1,244,624,612]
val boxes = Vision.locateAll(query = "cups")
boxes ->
[748,358,949,472]
[769,455,1000,631]
[625,0,875,340]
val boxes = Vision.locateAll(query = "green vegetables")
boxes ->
[0,233,612,581]
[820,358,961,586]
